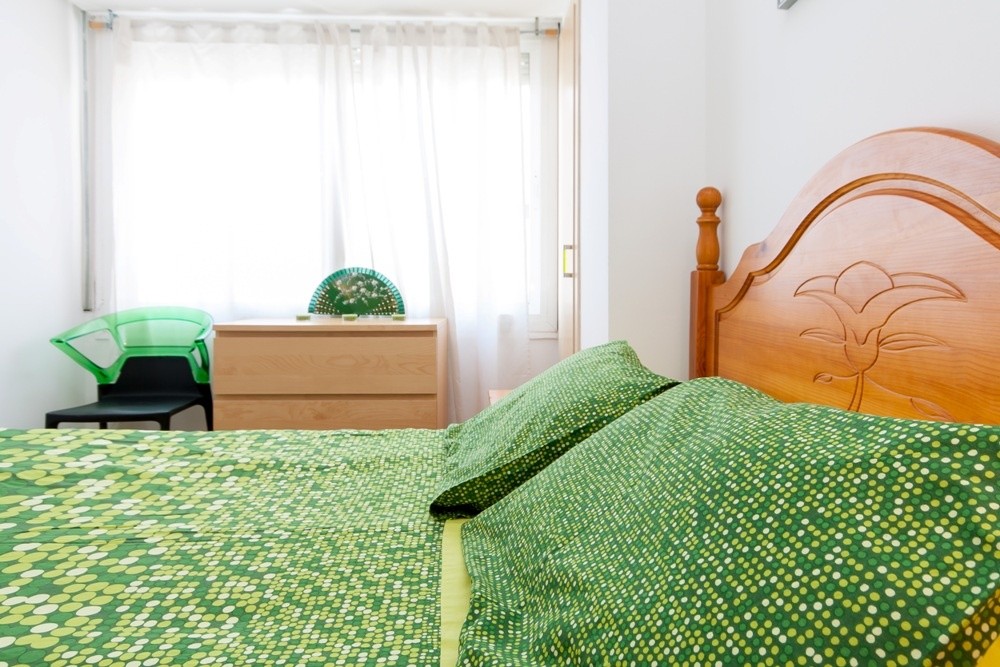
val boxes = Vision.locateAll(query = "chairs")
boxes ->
[42,309,213,432]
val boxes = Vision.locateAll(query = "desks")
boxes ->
[210,311,450,429]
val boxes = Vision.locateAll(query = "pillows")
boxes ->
[427,340,683,520]
[459,374,998,666]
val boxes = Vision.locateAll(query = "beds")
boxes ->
[0,128,999,666]
